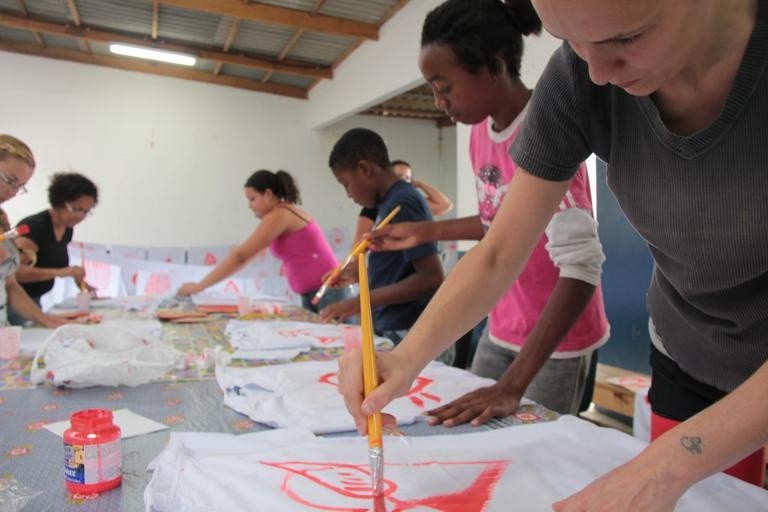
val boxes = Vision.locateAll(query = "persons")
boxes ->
[175,168,356,325]
[6,172,100,324]
[0,132,84,330]
[354,159,454,256]
[314,127,457,367]
[362,0,615,430]
[333,1,768,511]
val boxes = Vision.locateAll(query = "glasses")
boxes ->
[64,200,94,219]
[0,171,28,197]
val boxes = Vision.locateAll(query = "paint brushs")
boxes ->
[0,223,31,241]
[359,253,386,512]
[310,206,401,305]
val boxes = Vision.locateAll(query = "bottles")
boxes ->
[62,408,124,494]
